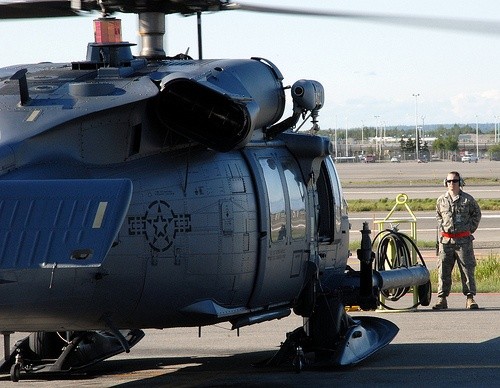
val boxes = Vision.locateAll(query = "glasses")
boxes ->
[446,179,459,184]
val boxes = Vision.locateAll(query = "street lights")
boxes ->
[374,116,380,152]
[413,93,420,158]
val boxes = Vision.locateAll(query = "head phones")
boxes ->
[444,177,464,187]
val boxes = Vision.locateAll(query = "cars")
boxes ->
[359,155,365,159]
[418,156,428,163]
[390,156,401,163]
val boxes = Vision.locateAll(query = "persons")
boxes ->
[432,171,481,310]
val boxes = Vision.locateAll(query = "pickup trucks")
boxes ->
[462,154,478,164]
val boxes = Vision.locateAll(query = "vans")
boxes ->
[366,155,375,163]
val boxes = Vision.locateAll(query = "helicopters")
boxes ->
[1,0,432,382]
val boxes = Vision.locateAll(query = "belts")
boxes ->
[441,230,471,238]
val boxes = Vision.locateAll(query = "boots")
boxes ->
[466,295,478,309]
[432,296,447,309]
